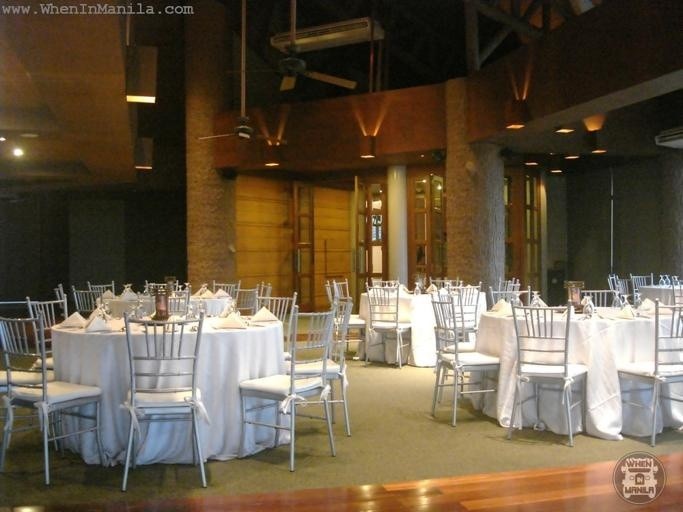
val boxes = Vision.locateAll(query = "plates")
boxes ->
[58,323,82,328]
[252,319,277,322]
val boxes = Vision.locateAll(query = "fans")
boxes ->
[269,0,360,93]
[186,0,277,145]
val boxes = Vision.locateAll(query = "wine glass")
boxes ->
[374,277,464,296]
[94,282,240,332]
[658,275,680,288]
[510,290,644,320]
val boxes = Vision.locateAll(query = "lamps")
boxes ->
[506,100,527,130]
[263,143,282,169]
[357,134,377,160]
[131,134,154,171]
[123,41,160,108]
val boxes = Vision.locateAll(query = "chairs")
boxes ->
[0,261,683,494]
